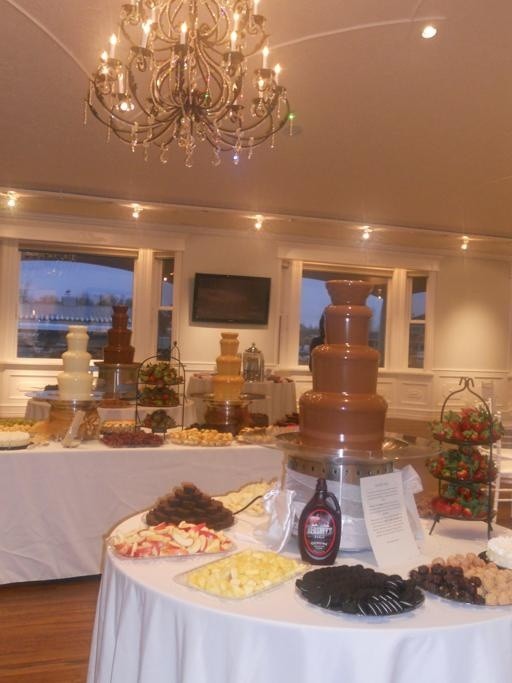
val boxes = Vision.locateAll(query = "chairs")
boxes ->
[454,397,512,528]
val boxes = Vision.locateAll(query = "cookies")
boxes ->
[295,563,421,617]
[146,479,235,530]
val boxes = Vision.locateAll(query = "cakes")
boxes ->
[485,536,512,569]
[0,430,30,449]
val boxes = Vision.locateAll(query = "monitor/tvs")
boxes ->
[192,273,270,324]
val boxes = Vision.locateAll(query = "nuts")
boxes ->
[408,552,512,606]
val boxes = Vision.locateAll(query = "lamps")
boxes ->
[81,0,296,169]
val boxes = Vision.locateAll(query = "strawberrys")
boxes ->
[425,406,502,521]
[138,361,180,430]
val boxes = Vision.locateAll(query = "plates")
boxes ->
[409,565,512,608]
[170,546,311,603]
[140,510,239,531]
[295,583,427,618]
[102,532,238,562]
[0,440,34,450]
[98,438,170,451]
[479,550,506,569]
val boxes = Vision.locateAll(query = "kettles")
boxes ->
[243,341,265,384]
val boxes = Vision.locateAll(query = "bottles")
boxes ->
[297,477,343,566]
